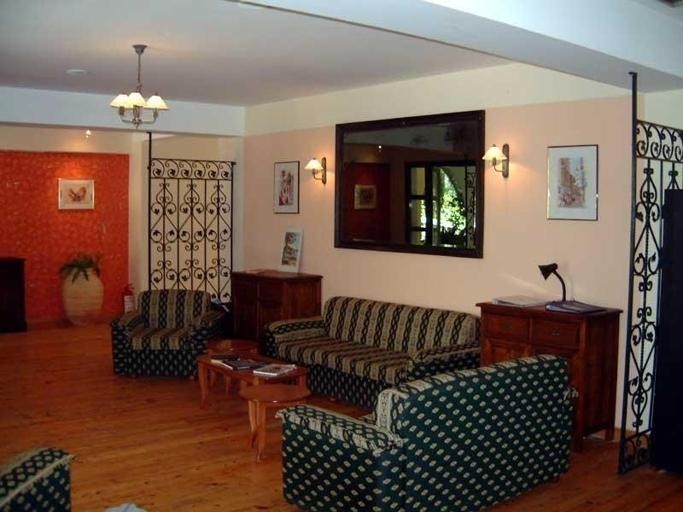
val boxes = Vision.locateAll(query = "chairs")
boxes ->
[0,446,78,511]
[111,289,224,380]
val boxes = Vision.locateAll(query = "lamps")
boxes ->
[538,263,566,302]
[482,143,509,178]
[304,157,326,184]
[110,44,168,129]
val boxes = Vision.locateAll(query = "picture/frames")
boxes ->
[547,145,599,221]
[354,184,377,210]
[57,178,94,210]
[277,228,303,272]
[273,161,299,214]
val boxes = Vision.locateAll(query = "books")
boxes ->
[209,353,240,366]
[221,358,260,371]
[252,362,299,380]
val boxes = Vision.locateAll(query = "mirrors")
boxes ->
[333,109,485,259]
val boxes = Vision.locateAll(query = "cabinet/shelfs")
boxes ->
[0,257,28,332]
[475,298,623,446]
[230,269,323,343]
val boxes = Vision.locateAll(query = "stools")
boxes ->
[239,384,311,463]
[207,339,258,396]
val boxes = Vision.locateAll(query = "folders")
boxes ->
[492,295,547,308]
[544,301,607,314]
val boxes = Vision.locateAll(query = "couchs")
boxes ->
[277,353,576,511]
[268,296,481,410]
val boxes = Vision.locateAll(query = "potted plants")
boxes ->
[56,255,103,323]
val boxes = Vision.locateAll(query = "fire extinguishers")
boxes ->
[121,283,136,315]
